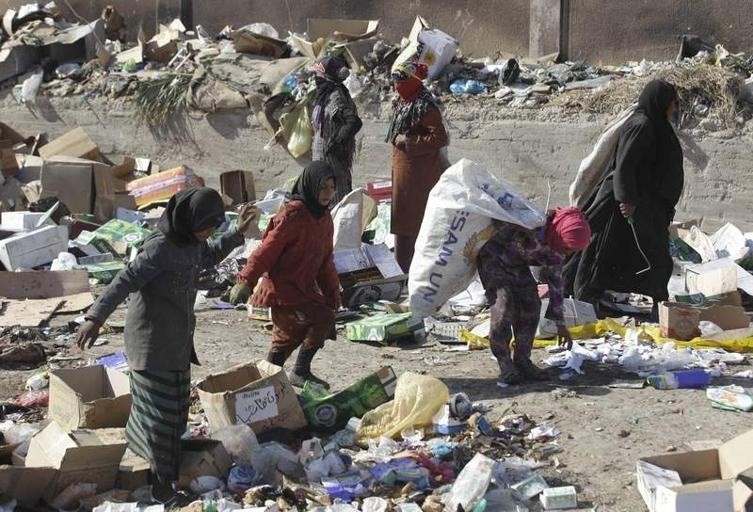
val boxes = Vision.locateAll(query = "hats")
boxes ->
[312,56,350,82]
[392,60,418,80]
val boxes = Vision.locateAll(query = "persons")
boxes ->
[477,206,591,384]
[74,186,257,504]
[385,60,449,287]
[230,160,342,390]
[561,79,682,322]
[312,56,363,210]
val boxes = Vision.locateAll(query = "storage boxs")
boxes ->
[198,353,400,440]
[391,14,461,81]
[137,22,186,66]
[220,170,256,203]
[1,225,66,273]
[637,426,752,511]
[328,244,407,301]
[38,156,113,220]
[0,17,106,80]
[0,353,134,512]
[1,208,46,233]
[662,219,752,342]
[37,125,99,160]
[126,165,206,210]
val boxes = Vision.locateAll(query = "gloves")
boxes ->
[229,282,251,307]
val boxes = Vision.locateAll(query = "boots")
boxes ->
[289,348,330,390]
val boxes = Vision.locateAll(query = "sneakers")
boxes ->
[498,367,521,385]
[517,363,549,381]
[148,482,192,508]
